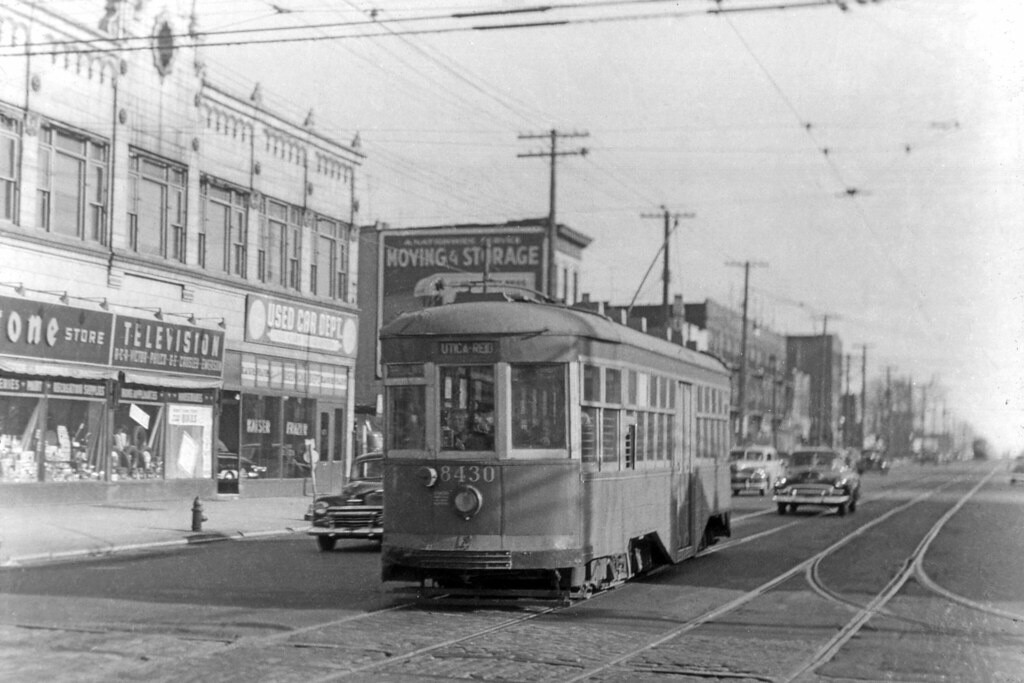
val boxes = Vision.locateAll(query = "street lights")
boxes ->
[768,354,778,449]
[739,320,760,444]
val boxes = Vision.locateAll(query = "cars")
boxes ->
[728,446,783,497]
[772,447,861,516]
[856,449,888,476]
[217,456,266,480]
[241,443,297,479]
[304,452,386,552]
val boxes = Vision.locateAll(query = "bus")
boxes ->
[380,293,732,606]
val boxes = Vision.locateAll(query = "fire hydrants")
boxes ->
[191,496,208,531]
